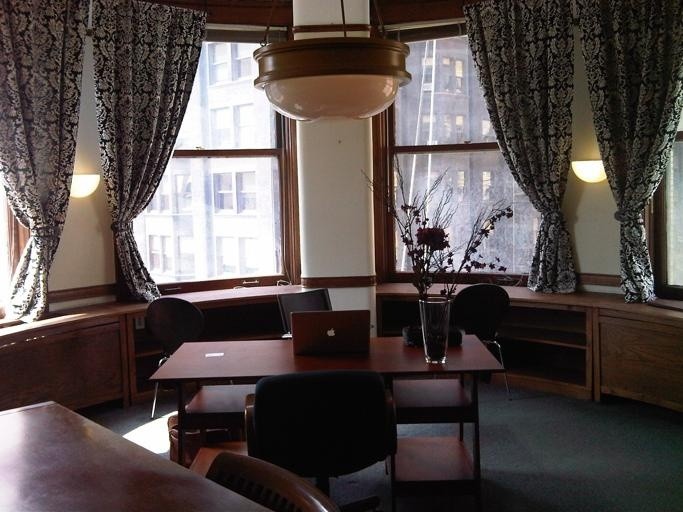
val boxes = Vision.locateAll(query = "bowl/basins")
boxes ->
[402,324,463,346]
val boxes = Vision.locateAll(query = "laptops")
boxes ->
[290,310,370,358]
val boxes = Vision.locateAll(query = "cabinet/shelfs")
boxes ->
[367,279,593,405]
[103,280,306,407]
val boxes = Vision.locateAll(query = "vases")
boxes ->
[414,295,451,364]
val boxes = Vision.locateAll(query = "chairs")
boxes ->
[239,361,404,512]
[200,448,341,512]
[142,295,237,422]
[449,280,517,404]
[275,286,333,338]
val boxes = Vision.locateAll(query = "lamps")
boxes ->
[570,156,609,183]
[66,171,102,198]
[245,1,415,125]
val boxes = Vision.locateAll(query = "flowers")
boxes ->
[358,143,520,351]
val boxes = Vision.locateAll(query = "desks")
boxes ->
[0,302,130,413]
[594,288,682,411]
[145,328,505,500]
[0,396,277,510]
